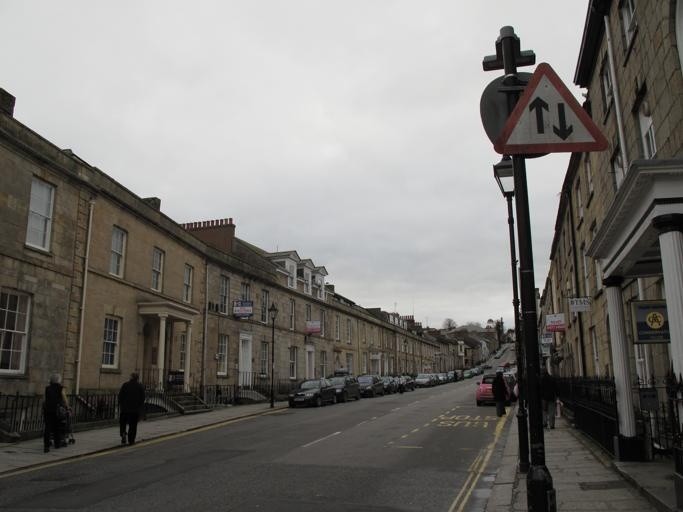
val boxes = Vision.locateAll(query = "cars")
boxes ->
[494,343,514,359]
[288,363,518,408]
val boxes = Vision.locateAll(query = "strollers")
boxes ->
[48,403,76,449]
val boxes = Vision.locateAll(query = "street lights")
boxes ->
[402,338,409,374]
[491,152,535,472]
[264,303,281,408]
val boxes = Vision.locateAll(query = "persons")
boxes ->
[43,374,69,452]
[492,372,509,417]
[454,372,458,381]
[541,368,558,429]
[117,372,146,444]
[461,371,464,380]
